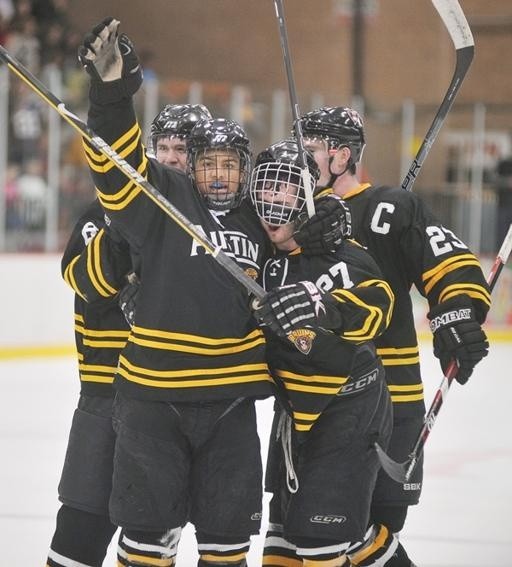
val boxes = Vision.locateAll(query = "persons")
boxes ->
[42,102,214,567]
[250,138,395,565]
[77,16,355,565]
[294,105,495,565]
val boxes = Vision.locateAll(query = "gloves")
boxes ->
[293,193,353,257]
[118,283,139,328]
[426,294,489,385]
[257,280,342,337]
[77,16,144,106]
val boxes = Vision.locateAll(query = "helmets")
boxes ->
[291,106,366,162]
[254,139,321,180]
[150,103,253,171]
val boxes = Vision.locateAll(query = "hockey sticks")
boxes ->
[376,221,512,489]
[400,0,477,203]
